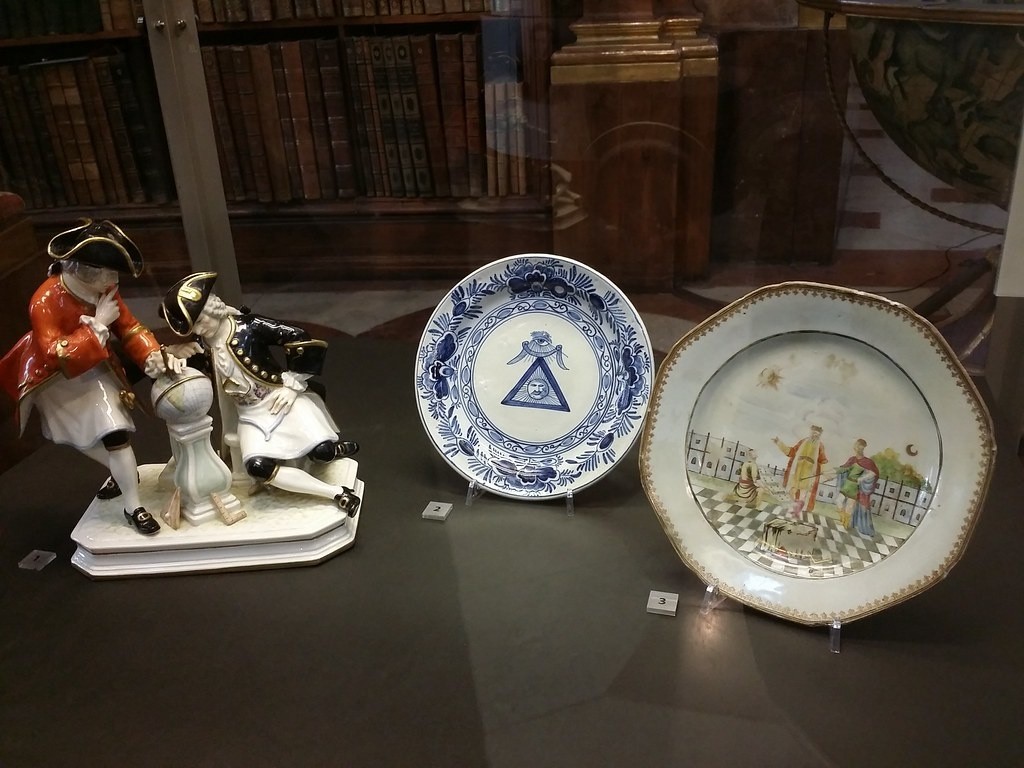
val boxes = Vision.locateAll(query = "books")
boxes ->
[0,0,528,210]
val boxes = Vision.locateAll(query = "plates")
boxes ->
[641,279,996,627]
[413,250,655,500]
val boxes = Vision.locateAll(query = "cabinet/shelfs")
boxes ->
[0,1,554,293]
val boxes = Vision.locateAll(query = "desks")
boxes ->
[0,306,1024,767]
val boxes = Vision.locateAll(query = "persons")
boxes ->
[158,271,361,518]
[0,217,187,534]
